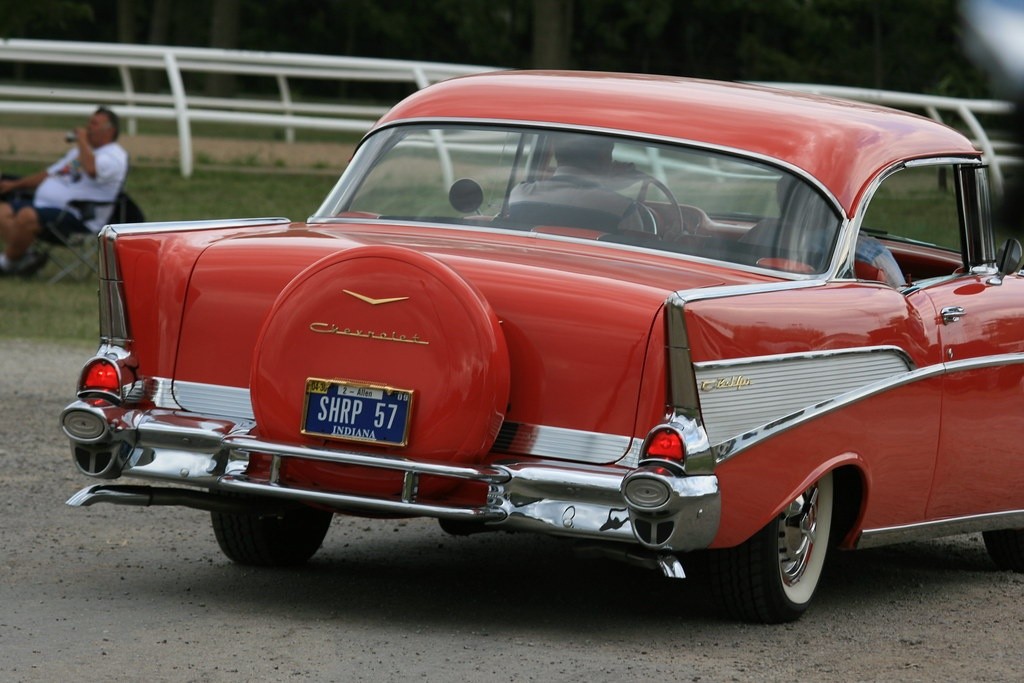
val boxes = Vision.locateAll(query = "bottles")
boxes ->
[63,131,91,144]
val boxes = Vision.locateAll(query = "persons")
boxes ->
[0,106,129,277]
[737,173,906,288]
[508,131,645,231]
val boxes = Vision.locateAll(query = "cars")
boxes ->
[59,70,1024,625]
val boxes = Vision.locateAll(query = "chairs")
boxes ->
[0,174,128,285]
[676,232,887,283]
[506,199,620,231]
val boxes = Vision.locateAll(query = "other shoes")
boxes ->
[0,261,37,285]
